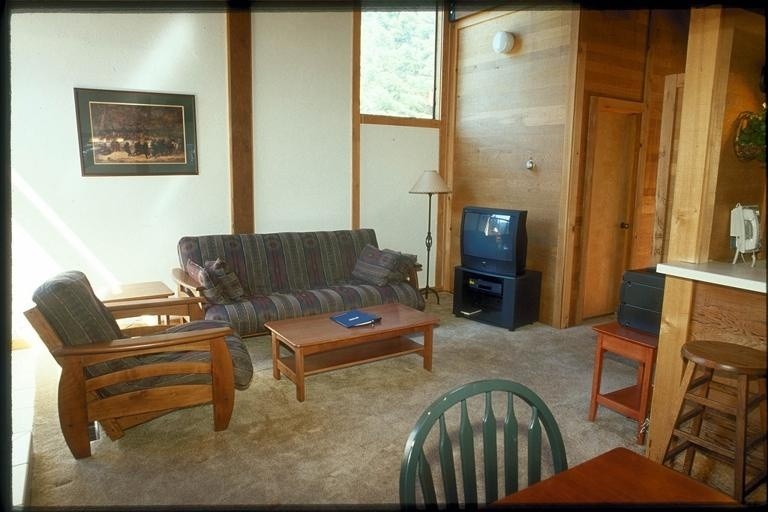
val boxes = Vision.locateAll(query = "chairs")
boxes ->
[399,378,568,511]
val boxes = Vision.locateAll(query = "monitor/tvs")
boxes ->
[460,206,528,278]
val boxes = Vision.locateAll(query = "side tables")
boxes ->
[103,280,174,326]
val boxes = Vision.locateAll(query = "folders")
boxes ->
[329,309,382,329]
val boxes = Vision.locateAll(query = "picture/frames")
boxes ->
[73,87,199,175]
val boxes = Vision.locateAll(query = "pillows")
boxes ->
[186,259,226,305]
[204,258,244,301]
[381,249,417,286]
[352,244,401,288]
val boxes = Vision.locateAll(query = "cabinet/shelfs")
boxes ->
[587,323,660,444]
[453,265,543,332]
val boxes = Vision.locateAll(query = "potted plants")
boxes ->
[734,109,768,168]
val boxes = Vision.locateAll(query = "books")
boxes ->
[460,307,482,316]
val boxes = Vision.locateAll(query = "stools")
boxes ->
[656,341,768,504]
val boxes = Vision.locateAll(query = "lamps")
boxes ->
[409,170,452,305]
[493,31,515,53]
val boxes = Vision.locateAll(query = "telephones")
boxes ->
[727,203,763,268]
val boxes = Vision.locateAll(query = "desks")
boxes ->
[264,303,440,402]
[489,446,746,507]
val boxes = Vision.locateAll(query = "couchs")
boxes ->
[171,228,425,339]
[24,271,253,459]
